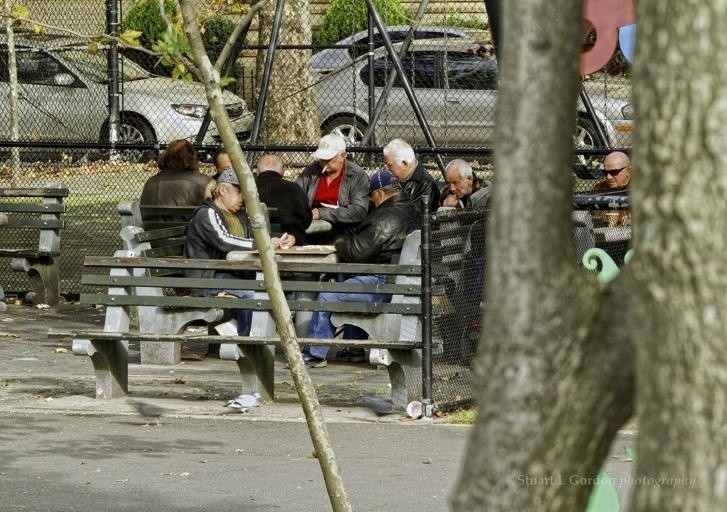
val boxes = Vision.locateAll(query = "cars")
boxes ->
[2,32,254,166]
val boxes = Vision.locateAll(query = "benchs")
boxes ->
[46,250,449,401]
[117,223,250,363]
[117,202,284,250]
[1,188,70,306]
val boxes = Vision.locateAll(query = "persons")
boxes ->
[140,131,492,369]
[467,40,497,64]
[590,151,632,226]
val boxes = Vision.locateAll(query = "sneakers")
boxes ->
[336,346,366,364]
[286,344,328,370]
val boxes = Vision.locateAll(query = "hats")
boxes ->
[314,134,346,161]
[216,167,241,187]
[362,170,399,198]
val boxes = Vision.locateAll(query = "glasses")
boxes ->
[601,164,628,176]
